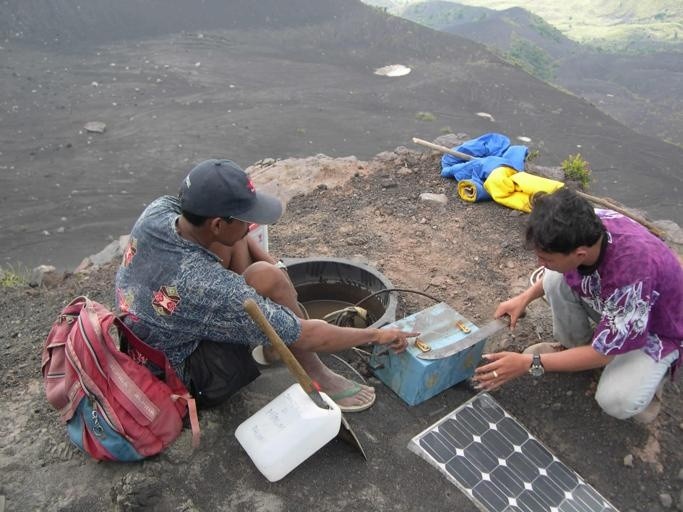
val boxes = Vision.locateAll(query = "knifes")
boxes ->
[417,311,527,361]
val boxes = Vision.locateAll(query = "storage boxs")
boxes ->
[368,301,488,408]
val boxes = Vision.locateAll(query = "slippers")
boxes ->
[330,380,376,413]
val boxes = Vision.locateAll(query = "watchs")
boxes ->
[528,352,545,378]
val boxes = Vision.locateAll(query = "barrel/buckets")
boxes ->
[235,381,342,482]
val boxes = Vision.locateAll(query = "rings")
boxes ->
[493,372,499,379]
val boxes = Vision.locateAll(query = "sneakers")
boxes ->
[522,341,570,355]
[633,366,669,423]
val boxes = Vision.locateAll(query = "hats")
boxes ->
[179,159,282,226]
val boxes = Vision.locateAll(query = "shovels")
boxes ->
[242,298,368,462]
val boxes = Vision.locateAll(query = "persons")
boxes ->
[473,185,683,420]
[114,158,422,413]
